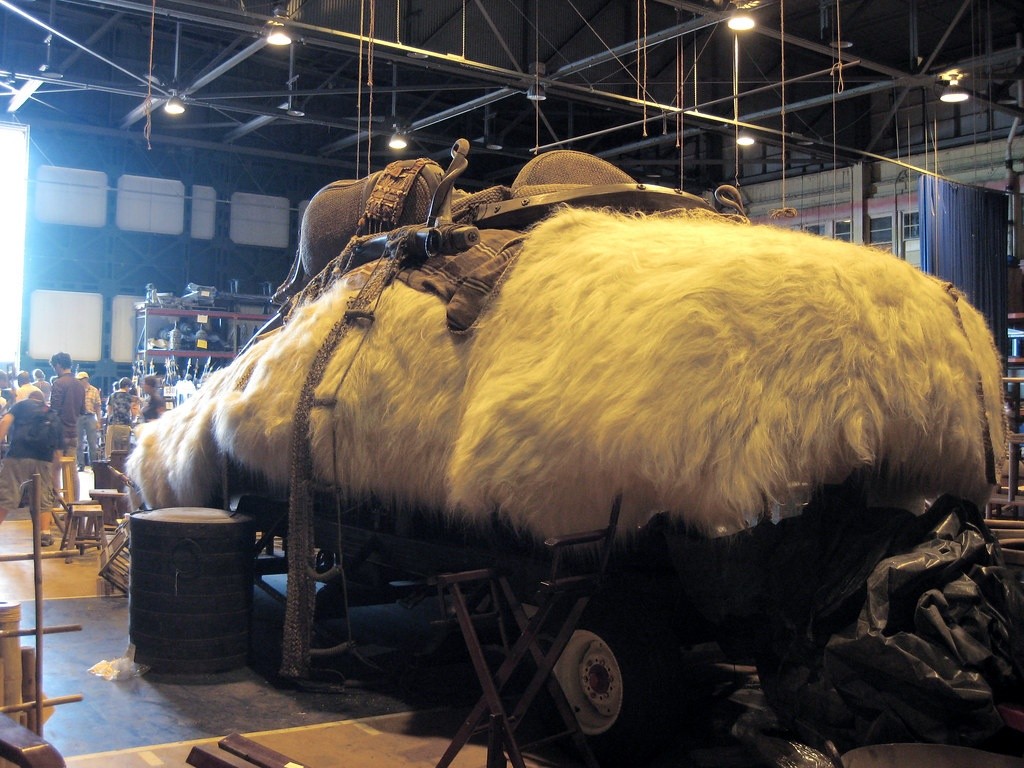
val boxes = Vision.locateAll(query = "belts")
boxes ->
[81,413,91,415]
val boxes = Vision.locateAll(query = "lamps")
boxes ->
[728,14,754,30]
[287,99,305,117]
[796,136,813,145]
[939,78,968,102]
[830,27,853,47]
[738,127,755,145]
[484,118,503,151]
[390,128,408,148]
[528,83,545,100]
[163,21,185,114]
[267,6,292,45]
[39,1,64,80]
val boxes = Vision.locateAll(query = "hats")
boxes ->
[16,371,30,379]
[76,371,89,380]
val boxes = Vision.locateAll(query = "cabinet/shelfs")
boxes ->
[134,307,274,425]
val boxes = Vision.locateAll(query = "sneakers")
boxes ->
[41,534,55,546]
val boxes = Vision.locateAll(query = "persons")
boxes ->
[0,352,169,546]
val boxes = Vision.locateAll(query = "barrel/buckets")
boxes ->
[129,508,256,686]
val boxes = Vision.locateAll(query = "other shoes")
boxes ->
[60,514,65,520]
[78,467,84,471]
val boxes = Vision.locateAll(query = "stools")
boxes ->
[61,505,107,563]
[86,489,119,534]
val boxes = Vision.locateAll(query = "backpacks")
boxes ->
[13,408,58,453]
